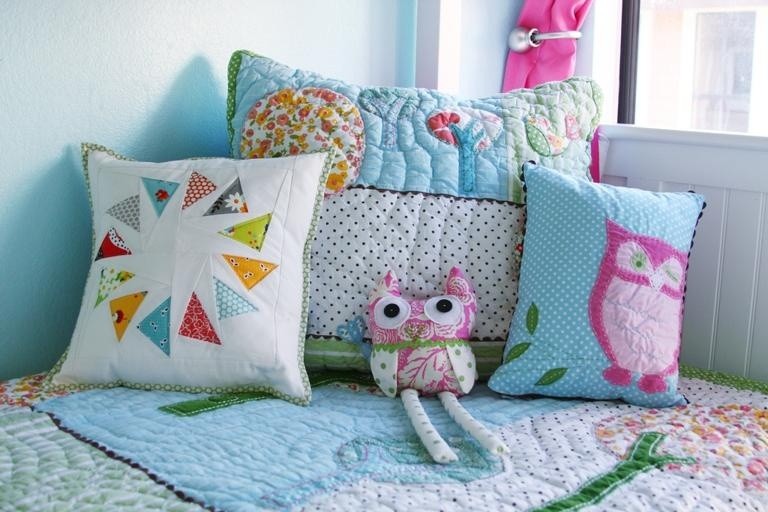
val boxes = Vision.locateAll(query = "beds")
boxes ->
[0,103,768,512]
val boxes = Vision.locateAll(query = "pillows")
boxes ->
[42,142,336,406]
[488,160,707,406]
[226,50,603,382]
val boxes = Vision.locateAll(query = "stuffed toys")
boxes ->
[362,266,510,467]
